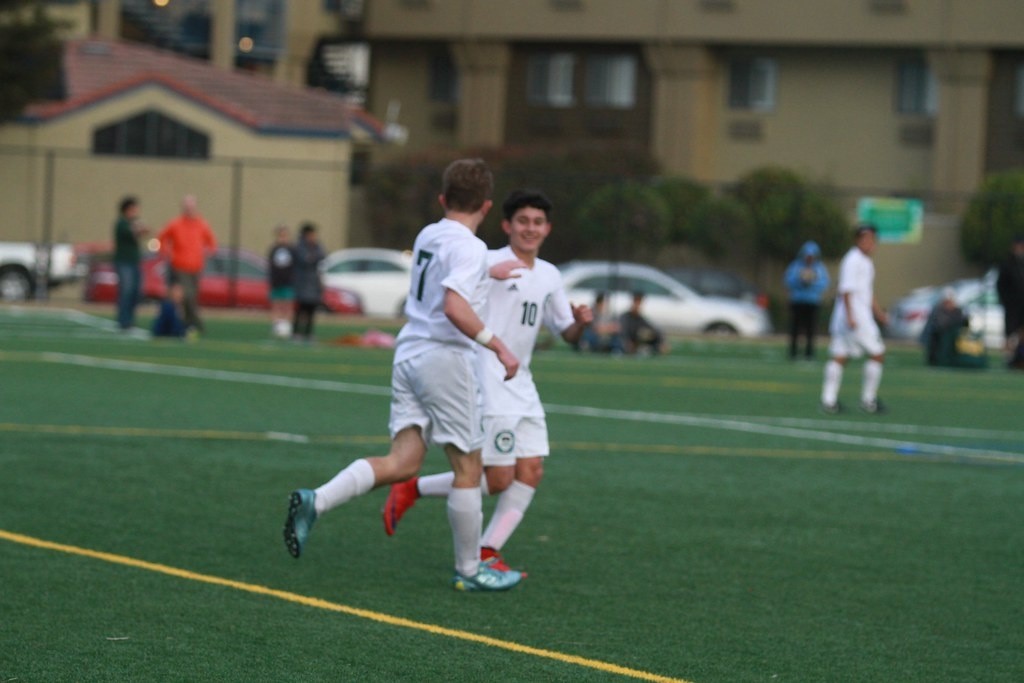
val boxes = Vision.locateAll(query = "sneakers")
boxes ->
[283,489,319,558]
[481,547,528,580]
[384,478,420,537]
[454,559,522,592]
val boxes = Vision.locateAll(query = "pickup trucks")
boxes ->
[0,242,78,303]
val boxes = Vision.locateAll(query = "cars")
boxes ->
[883,268,1004,347]
[86,242,361,314]
[316,248,414,320]
[557,261,774,338]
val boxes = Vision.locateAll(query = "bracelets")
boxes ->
[475,329,493,346]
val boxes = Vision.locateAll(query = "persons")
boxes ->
[785,239,828,358]
[268,221,325,338]
[159,196,215,332]
[382,189,593,579]
[820,226,888,416]
[113,198,152,327]
[282,157,528,591]
[997,237,1024,367]
[581,293,669,354]
[153,283,200,341]
[924,297,969,366]
